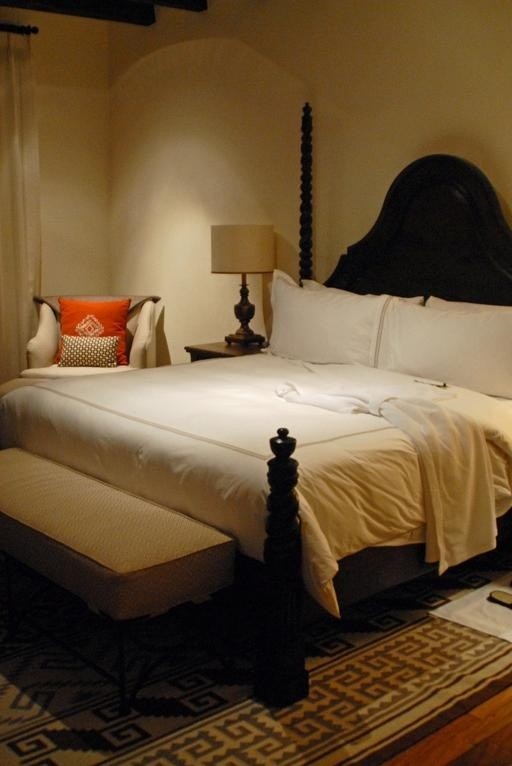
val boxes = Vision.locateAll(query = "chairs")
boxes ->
[27,294,164,366]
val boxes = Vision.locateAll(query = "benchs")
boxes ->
[2,447,241,709]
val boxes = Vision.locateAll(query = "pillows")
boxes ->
[267,267,512,395]
[53,297,132,367]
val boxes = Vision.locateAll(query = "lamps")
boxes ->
[210,224,276,349]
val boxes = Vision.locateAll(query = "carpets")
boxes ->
[2,557,512,766]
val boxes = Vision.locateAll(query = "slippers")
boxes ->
[491,590,512,606]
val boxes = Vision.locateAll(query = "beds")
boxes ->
[1,351,512,707]
